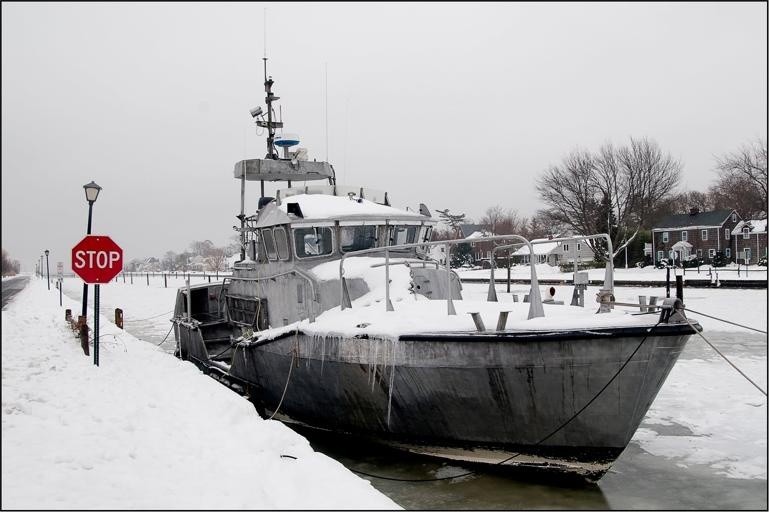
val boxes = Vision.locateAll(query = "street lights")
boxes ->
[80,181,102,317]
[36,250,50,290]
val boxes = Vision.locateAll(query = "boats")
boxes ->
[168,52,703,489]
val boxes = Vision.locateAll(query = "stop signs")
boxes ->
[71,235,123,284]
